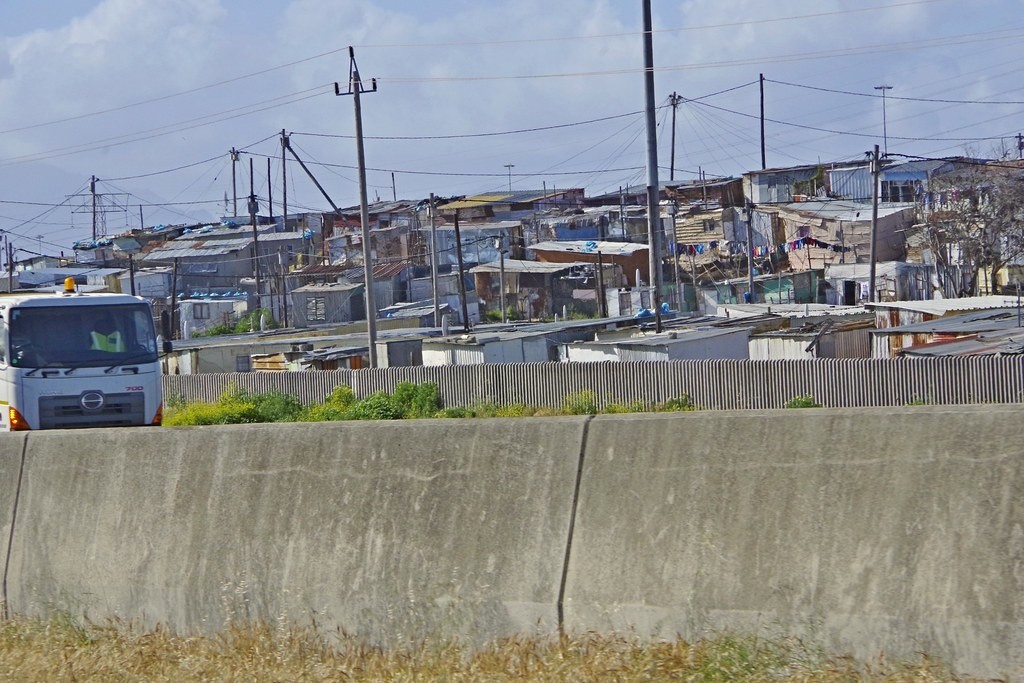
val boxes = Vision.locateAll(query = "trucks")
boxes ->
[1,278,172,432]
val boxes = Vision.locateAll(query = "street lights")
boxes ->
[874,85,893,159]
[35,233,44,255]
[504,164,515,198]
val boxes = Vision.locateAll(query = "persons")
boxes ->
[86,318,127,353]
